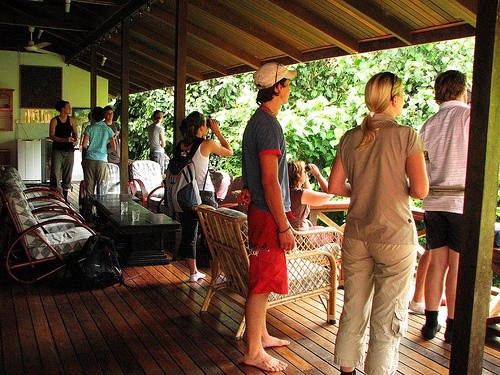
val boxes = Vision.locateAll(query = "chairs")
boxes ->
[0,168,98,285]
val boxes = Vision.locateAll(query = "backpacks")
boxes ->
[73,233,125,288]
[163,150,202,213]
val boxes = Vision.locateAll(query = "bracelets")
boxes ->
[279,227,291,233]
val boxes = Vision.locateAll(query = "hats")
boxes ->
[253,62,297,91]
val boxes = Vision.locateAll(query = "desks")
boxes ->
[88,194,181,265]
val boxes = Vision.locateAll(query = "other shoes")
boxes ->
[444,330,455,343]
[421,323,441,339]
[410,300,426,317]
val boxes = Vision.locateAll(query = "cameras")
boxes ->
[206,119,220,128]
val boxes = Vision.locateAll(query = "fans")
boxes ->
[24,27,57,55]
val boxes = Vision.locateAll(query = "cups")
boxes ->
[120,202,129,215]
[131,210,140,222]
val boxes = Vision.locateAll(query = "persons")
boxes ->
[173,111,233,284]
[420,71,470,343]
[82,107,117,221]
[243,62,298,371]
[103,106,121,164]
[50,101,78,205]
[409,246,431,315]
[329,72,429,375]
[147,110,166,174]
[287,159,344,289]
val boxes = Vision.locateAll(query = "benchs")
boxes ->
[192,205,336,341]
[219,177,244,208]
[148,171,231,214]
[129,160,165,209]
[79,162,120,208]
